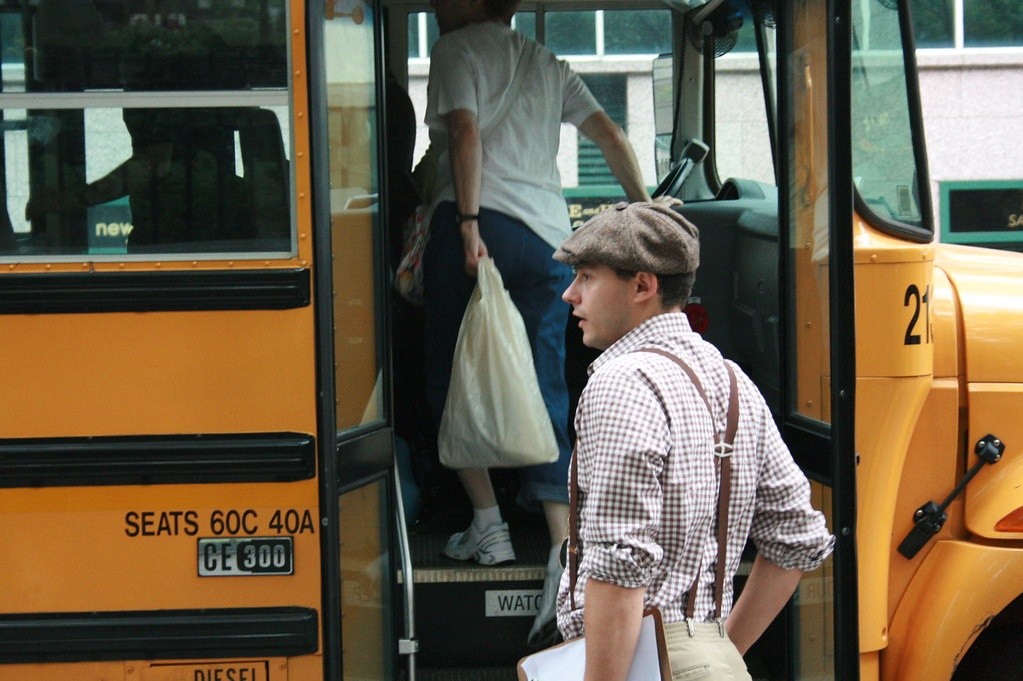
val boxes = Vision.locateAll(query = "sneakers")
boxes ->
[527,536,568,649]
[444,521,516,567]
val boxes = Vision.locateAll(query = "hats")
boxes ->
[551,201,700,275]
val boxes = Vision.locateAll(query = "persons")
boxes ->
[419,0,683,567]
[552,202,837,681]
[24,0,415,255]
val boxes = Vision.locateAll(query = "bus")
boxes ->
[0,0,1023,681]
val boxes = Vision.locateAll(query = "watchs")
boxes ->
[456,212,480,226]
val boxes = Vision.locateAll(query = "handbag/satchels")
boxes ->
[395,204,432,306]
[438,256,560,469]
